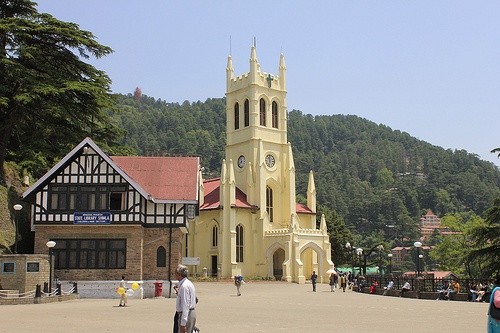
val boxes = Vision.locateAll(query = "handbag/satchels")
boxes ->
[490,289,500,319]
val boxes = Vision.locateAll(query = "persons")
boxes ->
[488,273,500,333]
[234,276,243,296]
[312,271,317,291]
[436,280,459,301]
[330,271,365,293]
[466,280,494,303]
[369,278,378,294]
[173,265,196,333]
[397,280,411,297]
[382,278,394,296]
[119,276,129,307]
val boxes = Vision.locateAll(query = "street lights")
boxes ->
[45,240,56,293]
[356,248,364,276]
[414,241,423,272]
[13,204,24,254]
[387,254,394,273]
[419,254,423,274]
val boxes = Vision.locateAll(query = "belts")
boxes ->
[180,308,194,313]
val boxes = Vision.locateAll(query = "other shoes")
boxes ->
[123,304,127,307]
[119,304,123,306]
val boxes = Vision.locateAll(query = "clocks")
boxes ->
[265,154,275,168]
[237,155,245,169]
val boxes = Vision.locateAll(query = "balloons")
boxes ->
[117,281,139,295]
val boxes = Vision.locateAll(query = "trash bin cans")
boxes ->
[153,282,164,297]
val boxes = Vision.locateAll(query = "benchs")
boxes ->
[448,292,469,301]
[351,285,400,297]
[475,291,492,302]
[418,292,440,300]
[400,290,417,298]
